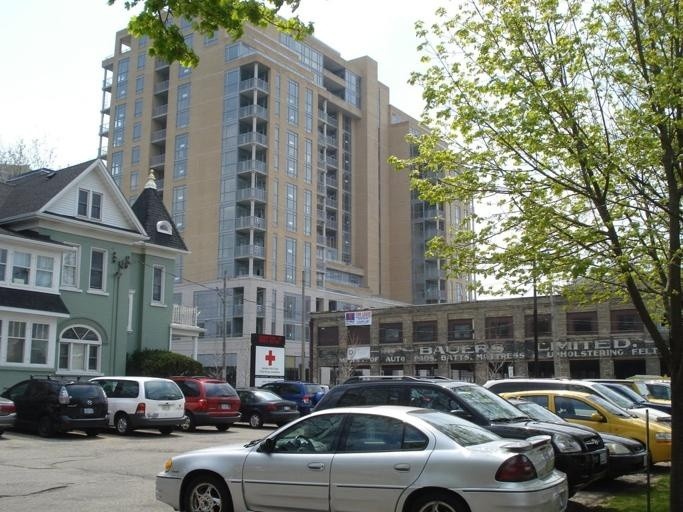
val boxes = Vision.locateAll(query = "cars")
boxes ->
[155,405,568,511]
[497,391,672,474]
[494,395,647,489]
[0,396,16,436]
[318,385,329,394]
[232,387,300,429]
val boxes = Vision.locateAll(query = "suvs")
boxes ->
[257,380,324,417]
[311,375,609,500]
[480,375,671,427]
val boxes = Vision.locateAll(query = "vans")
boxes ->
[86,376,187,436]
[162,375,241,433]
[0,375,110,438]
